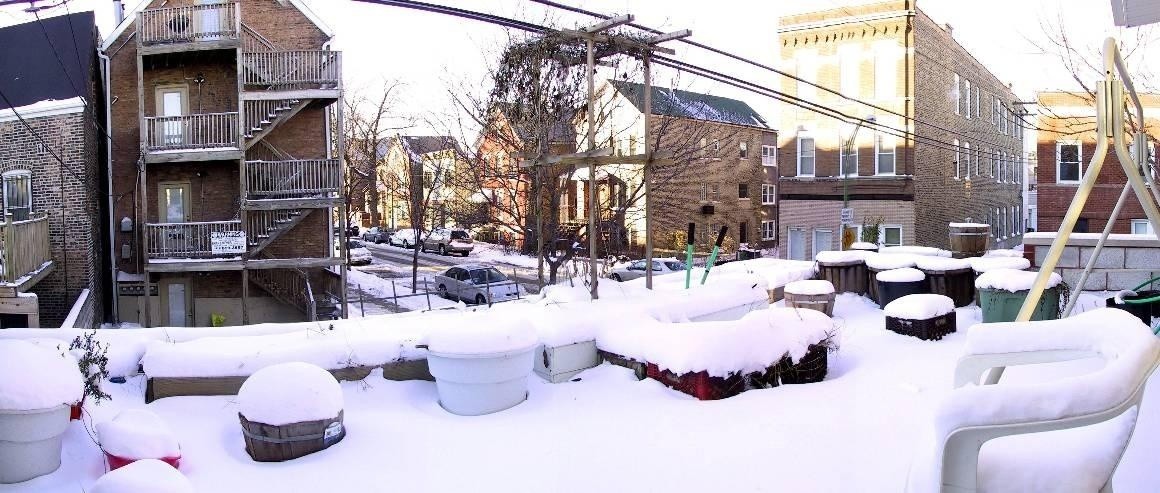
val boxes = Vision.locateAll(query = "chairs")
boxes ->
[919,305,1160,493]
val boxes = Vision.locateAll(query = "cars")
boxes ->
[435,263,525,305]
[332,219,426,266]
[606,254,687,281]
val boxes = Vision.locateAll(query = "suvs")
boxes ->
[420,225,473,256]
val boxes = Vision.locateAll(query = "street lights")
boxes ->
[842,115,877,234]
